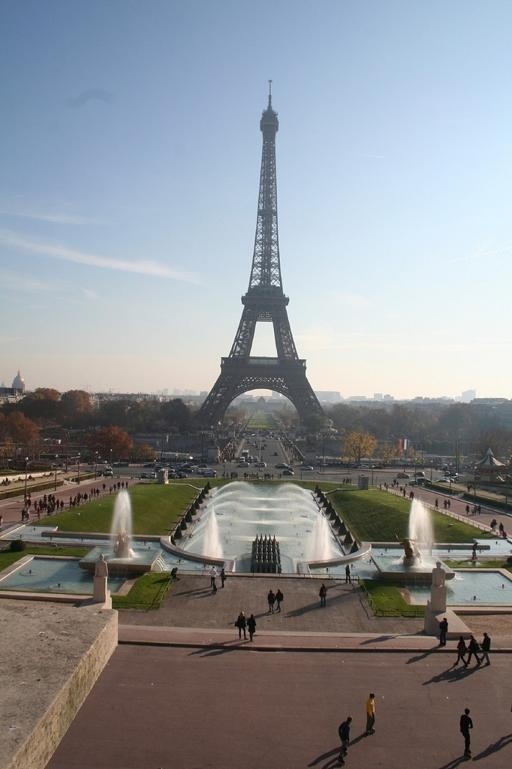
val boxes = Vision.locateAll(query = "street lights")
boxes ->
[463,459,479,503]
[23,455,28,500]
[160,429,170,463]
[201,431,204,462]
[77,452,80,486]
[94,450,98,480]
[53,454,58,490]
[175,447,178,463]
[109,448,112,468]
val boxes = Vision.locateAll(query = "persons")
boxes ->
[478,632,491,666]
[237,611,248,640]
[268,589,275,615]
[465,634,481,665]
[319,583,327,608]
[459,708,473,759]
[366,693,376,735]
[438,617,447,646]
[380,474,506,539]
[219,568,227,589]
[454,636,466,666]
[431,561,445,586]
[17,478,133,524]
[275,588,282,612]
[246,614,256,642]
[209,566,218,587]
[95,552,108,577]
[337,716,352,764]
[471,542,482,560]
[346,564,351,584]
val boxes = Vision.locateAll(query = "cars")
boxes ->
[237,430,315,476]
[150,451,218,479]
[347,461,384,469]
[395,460,459,485]
[104,469,113,476]
